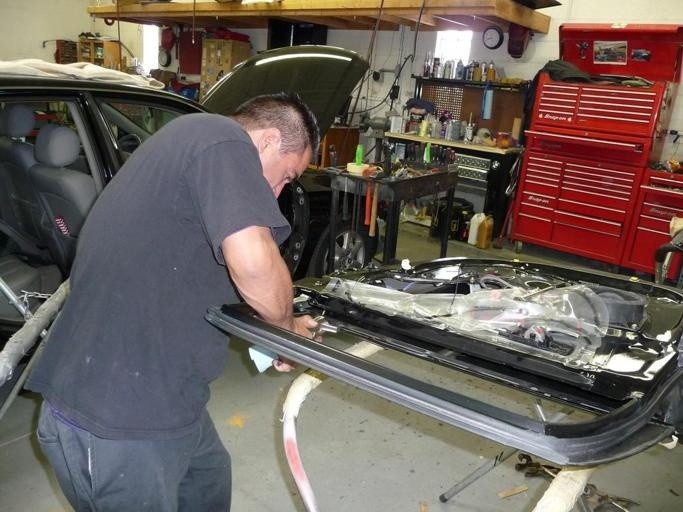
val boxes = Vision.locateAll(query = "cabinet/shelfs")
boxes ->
[307,129,359,170]
[199,39,250,107]
[78,40,120,71]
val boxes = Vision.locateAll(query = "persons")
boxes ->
[24,92,322,512]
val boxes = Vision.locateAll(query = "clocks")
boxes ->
[482,26,504,49]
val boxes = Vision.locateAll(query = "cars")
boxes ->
[0,44,384,376]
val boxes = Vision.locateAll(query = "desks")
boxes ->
[329,161,458,265]
[383,132,524,241]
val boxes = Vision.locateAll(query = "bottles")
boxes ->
[497,132,510,148]
[355,144,362,165]
[422,50,496,81]
[405,115,423,134]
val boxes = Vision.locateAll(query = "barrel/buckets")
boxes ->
[431,196,494,248]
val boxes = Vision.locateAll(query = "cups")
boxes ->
[418,120,442,139]
[445,126,454,139]
